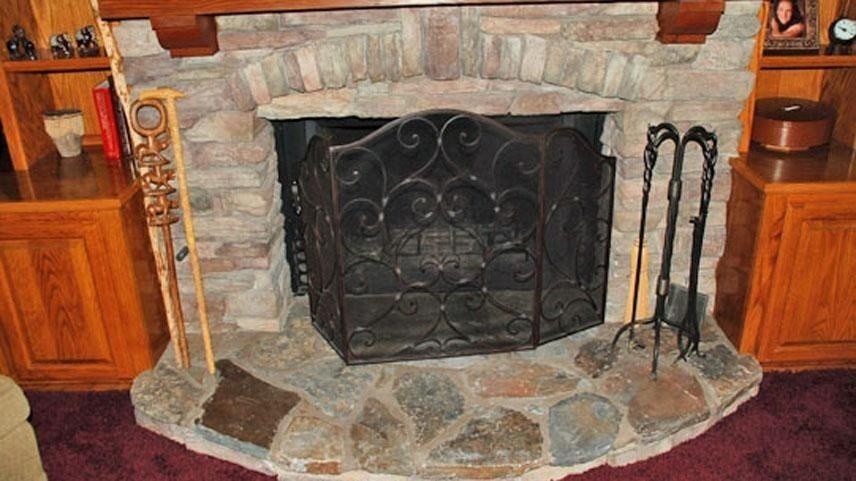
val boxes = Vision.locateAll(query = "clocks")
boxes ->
[826,17,856,55]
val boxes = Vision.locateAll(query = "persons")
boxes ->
[769,1,805,38]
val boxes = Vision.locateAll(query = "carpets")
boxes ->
[25,369,856,481]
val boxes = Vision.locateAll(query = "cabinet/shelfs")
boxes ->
[0,0,171,393]
[715,0,856,372]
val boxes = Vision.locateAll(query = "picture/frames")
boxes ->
[764,0,819,54]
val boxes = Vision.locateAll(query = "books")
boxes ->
[94,75,134,161]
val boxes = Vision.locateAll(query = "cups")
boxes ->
[41,111,86,157]
